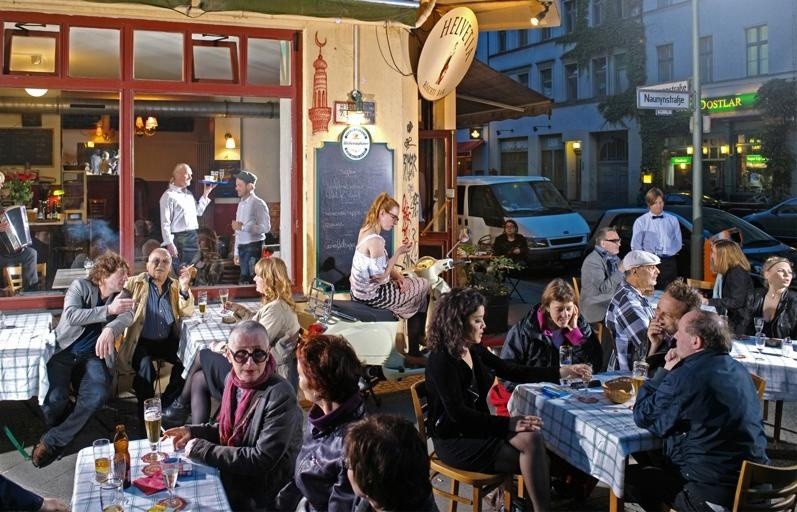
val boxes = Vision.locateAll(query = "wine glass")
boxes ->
[218,288,229,314]
[197,291,208,323]
[582,364,593,399]
[559,345,573,384]
[754,316,765,339]
[108,454,128,505]
[755,332,766,360]
[160,457,179,506]
[632,361,649,400]
[219,169,224,182]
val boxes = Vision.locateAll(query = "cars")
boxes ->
[739,197,797,237]
[582,205,796,287]
[663,191,727,210]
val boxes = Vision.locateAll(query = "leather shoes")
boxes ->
[404,354,427,368]
[32,437,53,468]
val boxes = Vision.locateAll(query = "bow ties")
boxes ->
[652,215,663,218]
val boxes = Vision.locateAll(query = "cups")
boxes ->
[92,439,111,484]
[210,170,218,182]
[144,398,162,459]
[99,478,124,512]
[204,175,214,181]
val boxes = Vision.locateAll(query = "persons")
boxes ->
[159,163,218,276]
[499,278,603,511]
[30,229,52,262]
[699,239,797,340]
[348,193,437,347]
[605,250,662,374]
[159,321,303,512]
[0,171,5,192]
[84,143,119,177]
[491,220,529,289]
[578,226,627,372]
[144,217,162,244]
[180,226,223,288]
[276,336,440,512]
[230,170,271,285]
[132,220,149,243]
[72,231,110,267]
[134,239,175,275]
[160,257,300,437]
[624,279,772,512]
[31,248,197,469]
[0,210,40,291]
[631,188,683,291]
[426,288,593,512]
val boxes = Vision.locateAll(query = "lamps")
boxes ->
[350,90,365,113]
[529,1,552,27]
[224,131,236,150]
[144,116,159,136]
[135,115,144,136]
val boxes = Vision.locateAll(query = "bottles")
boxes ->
[781,336,793,358]
[113,425,132,488]
[36,200,57,221]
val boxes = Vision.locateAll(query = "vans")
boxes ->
[454,175,590,264]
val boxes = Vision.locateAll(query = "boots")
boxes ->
[161,397,190,426]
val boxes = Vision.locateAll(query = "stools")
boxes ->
[311,309,400,409]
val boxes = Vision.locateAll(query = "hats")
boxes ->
[233,171,258,184]
[623,250,662,271]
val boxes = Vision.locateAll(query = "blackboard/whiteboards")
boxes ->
[316,125,395,293]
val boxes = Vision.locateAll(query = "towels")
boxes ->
[132,470,178,496]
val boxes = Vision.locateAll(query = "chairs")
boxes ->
[2,261,47,297]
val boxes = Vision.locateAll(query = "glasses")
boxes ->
[605,239,620,242]
[228,347,270,363]
[388,211,400,220]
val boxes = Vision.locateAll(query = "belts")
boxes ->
[238,241,264,247]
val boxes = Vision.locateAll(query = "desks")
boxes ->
[459,252,498,271]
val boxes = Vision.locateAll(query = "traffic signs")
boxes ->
[636,79,689,92]
[636,87,690,110]
